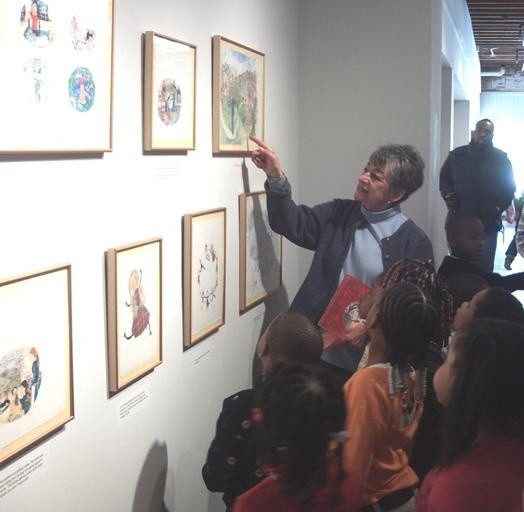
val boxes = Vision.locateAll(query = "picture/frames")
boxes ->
[212,35,266,155]
[182,208,226,347]
[144,30,196,152]
[104,237,163,397]
[0,263,74,464]
[238,191,282,314]
[0,1,114,156]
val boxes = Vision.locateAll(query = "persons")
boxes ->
[203,258,523,512]
[249,136,433,374]
[439,119,515,272]
[505,202,524,270]
[437,214,524,292]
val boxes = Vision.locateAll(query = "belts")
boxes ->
[360,486,414,511]
